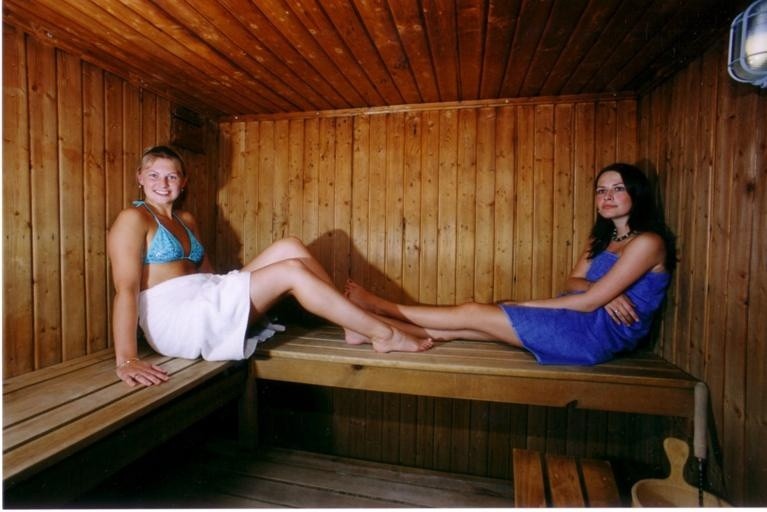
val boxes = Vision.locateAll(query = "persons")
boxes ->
[344,162,676,365]
[106,146,434,387]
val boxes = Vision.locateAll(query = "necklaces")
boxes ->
[612,227,635,241]
[172,220,183,237]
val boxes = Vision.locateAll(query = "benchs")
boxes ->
[2,342,249,509]
[509,443,623,508]
[250,329,700,450]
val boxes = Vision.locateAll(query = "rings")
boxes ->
[615,310,617,313]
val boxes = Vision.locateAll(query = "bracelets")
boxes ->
[115,358,138,369]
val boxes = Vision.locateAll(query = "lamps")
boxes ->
[725,0,766,88]
